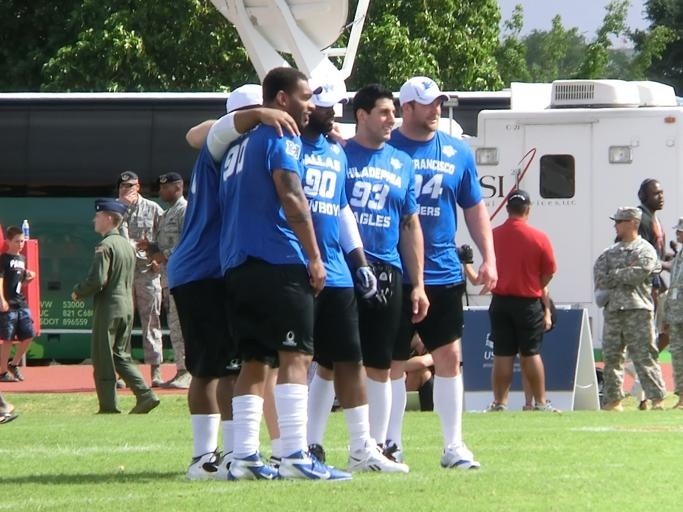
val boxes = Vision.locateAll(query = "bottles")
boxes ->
[21,219,30,241]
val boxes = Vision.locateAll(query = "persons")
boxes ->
[70,196,160,414]
[0,225,36,382]
[593,205,668,411]
[167,68,497,479]
[661,217,683,409]
[0,392,18,424]
[614,178,670,411]
[485,189,558,411]
[520,285,552,410]
[116,170,163,390]
[135,172,193,389]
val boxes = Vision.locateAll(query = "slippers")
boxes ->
[0,412,18,424]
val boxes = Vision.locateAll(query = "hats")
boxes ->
[226,75,451,113]
[508,189,531,205]
[608,206,642,223]
[95,171,183,216]
[671,217,683,231]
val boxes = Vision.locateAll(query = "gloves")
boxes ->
[354,265,379,301]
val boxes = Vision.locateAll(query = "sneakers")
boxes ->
[92,365,192,413]
[7,361,24,381]
[0,371,21,382]
[184,442,482,482]
[483,391,683,415]
